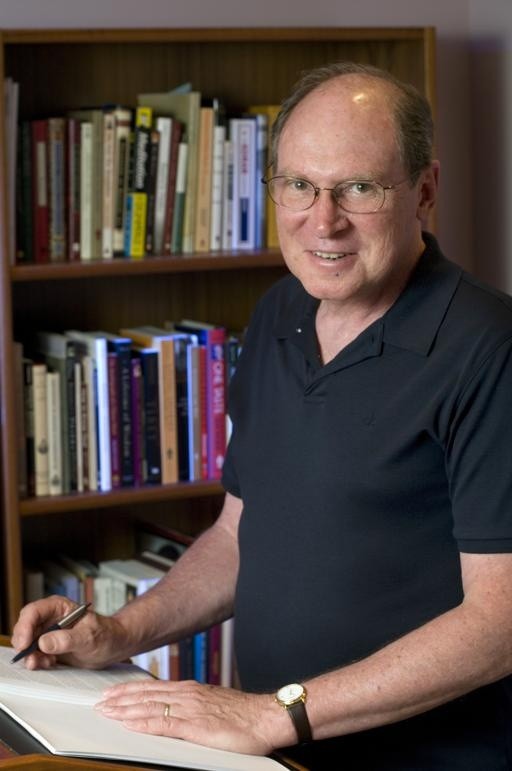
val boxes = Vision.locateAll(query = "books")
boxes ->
[0,643,298,771]
[22,523,236,688]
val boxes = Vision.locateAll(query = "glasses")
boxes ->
[260,160,428,215]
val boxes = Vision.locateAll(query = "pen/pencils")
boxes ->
[10,603,92,665]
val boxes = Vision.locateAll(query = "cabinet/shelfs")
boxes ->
[0,23,440,640]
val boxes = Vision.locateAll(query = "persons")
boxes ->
[13,60,511,771]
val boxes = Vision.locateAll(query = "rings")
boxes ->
[164,702,170,717]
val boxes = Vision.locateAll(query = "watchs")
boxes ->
[276,681,313,746]
